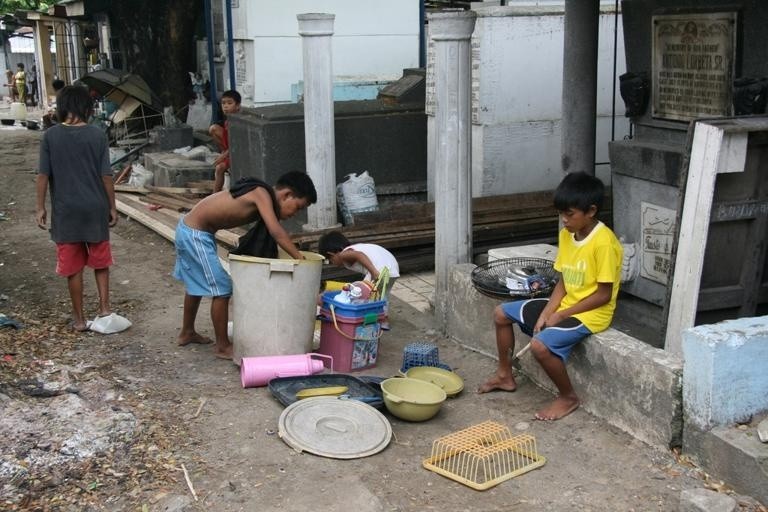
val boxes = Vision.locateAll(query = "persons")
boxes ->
[318,230,400,332]
[42,81,65,128]
[478,171,624,420]
[208,90,242,195]
[12,63,26,102]
[30,60,39,106]
[37,86,118,333]
[173,170,318,360]
[2,70,29,102]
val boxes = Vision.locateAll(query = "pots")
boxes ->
[378,377,447,423]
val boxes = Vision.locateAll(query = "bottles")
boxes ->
[332,285,350,304]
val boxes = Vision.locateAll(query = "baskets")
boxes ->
[397,342,454,380]
[422,420,548,491]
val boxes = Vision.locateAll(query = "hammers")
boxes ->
[507,326,546,369]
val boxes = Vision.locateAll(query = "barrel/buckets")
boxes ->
[11,102,27,120]
[314,290,388,373]
[229,251,326,367]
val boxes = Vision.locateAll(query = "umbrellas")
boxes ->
[79,69,165,129]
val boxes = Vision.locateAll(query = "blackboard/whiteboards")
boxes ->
[651,11,740,123]
